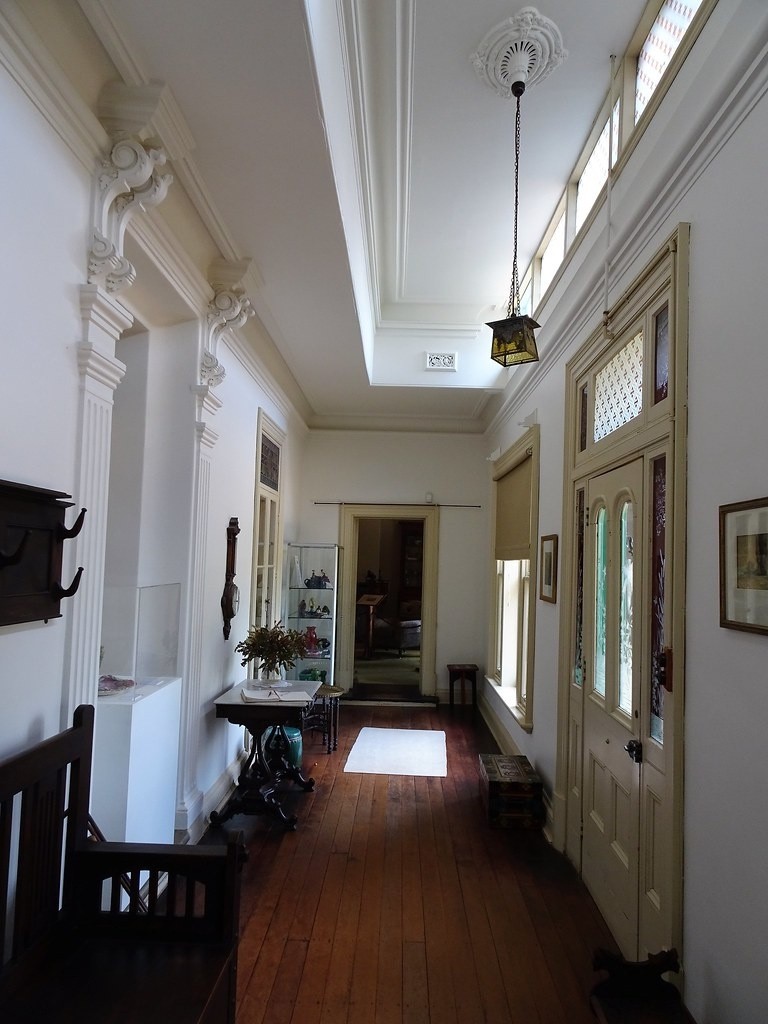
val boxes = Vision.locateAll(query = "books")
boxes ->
[241,689,314,702]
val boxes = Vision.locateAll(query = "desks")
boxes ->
[314,685,345,755]
[210,679,324,832]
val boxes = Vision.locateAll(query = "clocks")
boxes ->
[221,517,240,640]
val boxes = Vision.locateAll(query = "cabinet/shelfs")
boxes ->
[287,542,339,688]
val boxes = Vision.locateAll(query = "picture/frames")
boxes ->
[539,534,559,604]
[718,496,768,636]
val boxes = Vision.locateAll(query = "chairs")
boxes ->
[0,705,252,1024]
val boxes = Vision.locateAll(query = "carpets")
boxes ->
[342,725,448,778]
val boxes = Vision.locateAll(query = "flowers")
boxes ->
[233,621,309,673]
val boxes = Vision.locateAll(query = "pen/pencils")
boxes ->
[268,692,271,697]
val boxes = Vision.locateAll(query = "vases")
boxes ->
[261,657,281,680]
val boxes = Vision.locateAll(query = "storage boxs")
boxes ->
[480,753,545,832]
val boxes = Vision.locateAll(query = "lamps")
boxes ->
[485,81,543,367]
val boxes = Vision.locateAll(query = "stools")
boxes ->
[447,664,480,718]
